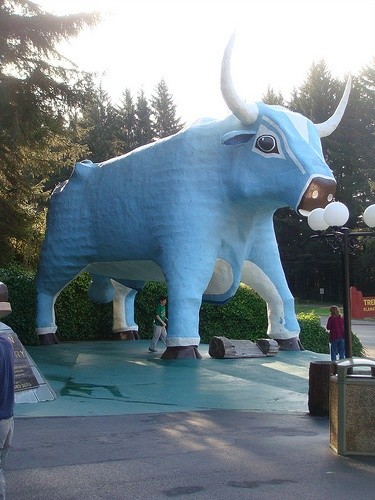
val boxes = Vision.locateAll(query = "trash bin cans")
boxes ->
[329,358,375,456]
[308,361,337,417]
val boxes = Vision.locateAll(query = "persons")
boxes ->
[148,297,168,352]
[326,305,345,362]
[0,333,15,500]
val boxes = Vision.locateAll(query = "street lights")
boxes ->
[305,197,374,357]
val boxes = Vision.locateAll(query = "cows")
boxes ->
[35,33,352,359]
